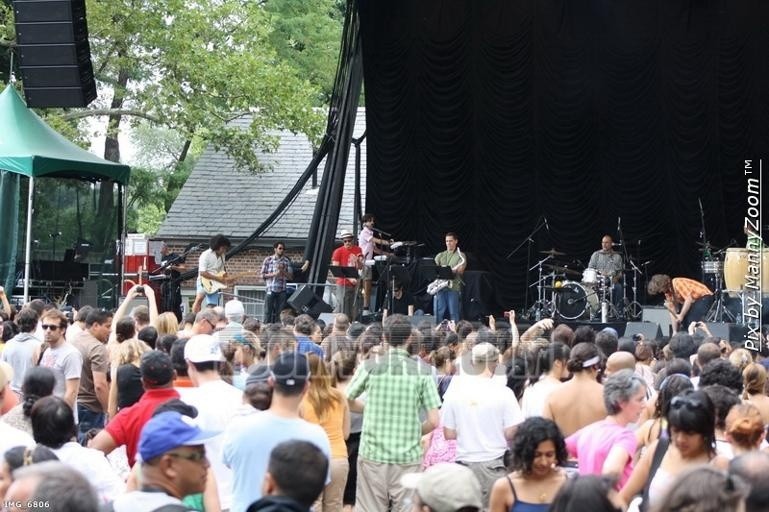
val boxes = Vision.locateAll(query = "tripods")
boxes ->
[704,274,736,323]
[527,254,642,322]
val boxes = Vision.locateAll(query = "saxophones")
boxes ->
[426,247,464,295]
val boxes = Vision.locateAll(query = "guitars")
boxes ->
[200,270,257,294]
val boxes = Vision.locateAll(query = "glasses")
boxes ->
[42,324,60,330]
[671,396,703,410]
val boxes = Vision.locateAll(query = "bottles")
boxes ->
[536,308,540,321]
[144,257,149,271]
[408,305,414,316]
[601,302,608,323]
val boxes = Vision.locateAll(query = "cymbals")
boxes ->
[543,263,580,275]
[545,286,571,293]
[539,250,566,256]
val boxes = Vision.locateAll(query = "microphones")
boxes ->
[48,232,62,238]
[612,243,622,246]
[544,218,550,232]
[363,223,373,229]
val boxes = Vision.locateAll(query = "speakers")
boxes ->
[12,0,97,107]
[318,312,340,325]
[408,316,434,329]
[624,323,663,341]
[697,324,753,344]
[287,284,333,321]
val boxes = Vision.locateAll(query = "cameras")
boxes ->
[441,319,452,326]
[504,312,511,318]
[484,316,496,326]
[136,286,145,293]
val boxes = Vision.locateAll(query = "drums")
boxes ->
[583,268,597,284]
[724,247,769,298]
[553,281,598,320]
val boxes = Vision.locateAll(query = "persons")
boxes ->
[587,235,625,319]
[647,274,715,332]
[357,212,396,316]
[331,229,365,322]
[270,258,310,323]
[0,281,768,511]
[196,233,235,308]
[261,241,294,327]
[433,232,466,323]
[158,242,187,324]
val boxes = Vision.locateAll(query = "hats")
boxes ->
[341,232,354,240]
[135,305,150,321]
[472,342,499,362]
[141,333,225,459]
[404,462,481,510]
